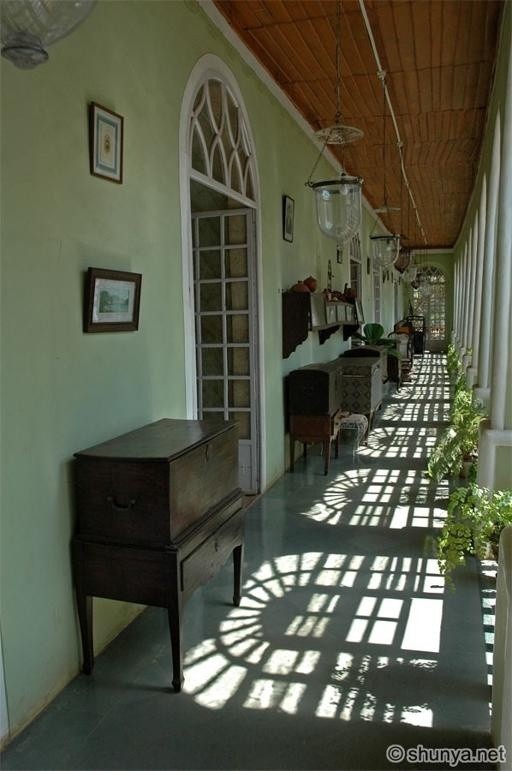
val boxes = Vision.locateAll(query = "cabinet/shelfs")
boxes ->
[70,416,246,697]
[283,288,427,480]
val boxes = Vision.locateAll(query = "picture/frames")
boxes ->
[282,194,296,245]
[88,99,125,188]
[81,264,144,337]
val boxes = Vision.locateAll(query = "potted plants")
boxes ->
[424,482,512,594]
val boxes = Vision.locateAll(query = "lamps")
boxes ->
[303,2,366,247]
[0,0,98,72]
[362,68,423,290]
